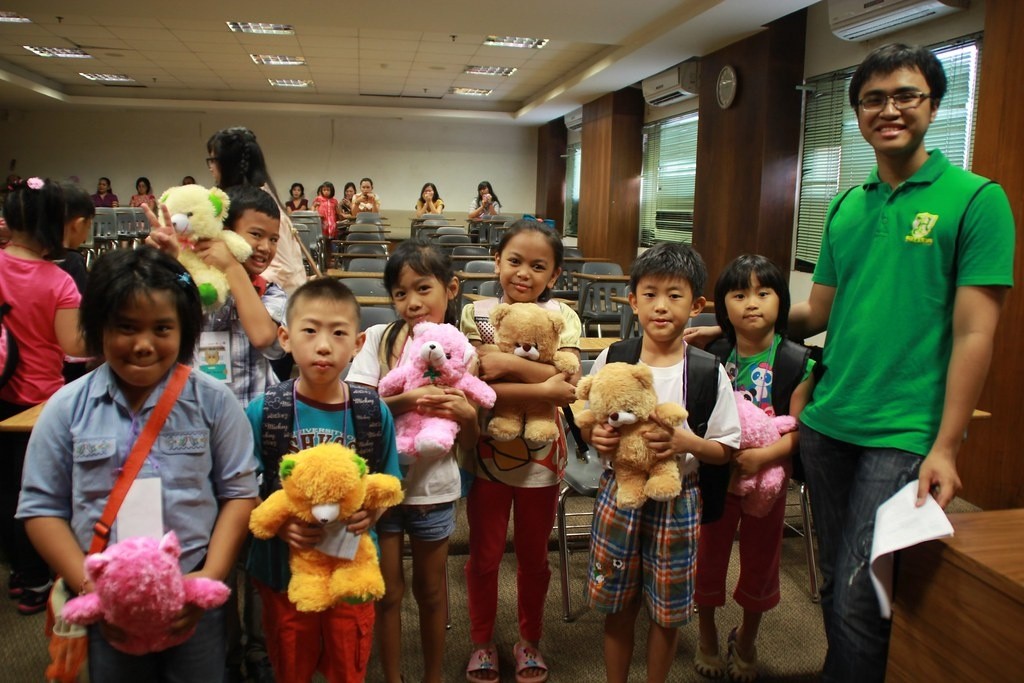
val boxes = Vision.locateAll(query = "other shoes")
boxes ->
[694,631,724,679]
[724,624,759,683]
[6,567,54,613]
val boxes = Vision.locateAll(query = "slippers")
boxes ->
[465,642,500,683]
[513,640,549,683]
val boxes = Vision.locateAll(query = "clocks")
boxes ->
[715,64,737,109]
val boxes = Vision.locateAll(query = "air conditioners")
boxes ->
[642,60,701,108]
[563,106,582,132]
[827,0,970,44]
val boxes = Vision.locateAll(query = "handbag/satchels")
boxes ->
[0,304,20,387]
[43,569,91,683]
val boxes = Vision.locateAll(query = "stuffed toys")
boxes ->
[726,390,800,517]
[574,362,689,511]
[248,443,406,612]
[156,184,254,310]
[61,529,231,656]
[378,320,497,465]
[481,302,580,444]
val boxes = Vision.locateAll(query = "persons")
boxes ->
[467,181,500,243]
[244,277,404,683]
[345,237,481,683]
[584,240,740,683]
[139,189,290,409]
[15,244,259,683]
[694,253,817,683]
[0,175,86,614]
[681,40,1015,683]
[43,182,97,384]
[90,126,381,298]
[460,219,584,683]
[415,182,444,229]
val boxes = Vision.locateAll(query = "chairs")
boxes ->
[76,202,821,622]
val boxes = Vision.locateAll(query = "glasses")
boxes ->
[205,155,219,171]
[855,90,937,112]
